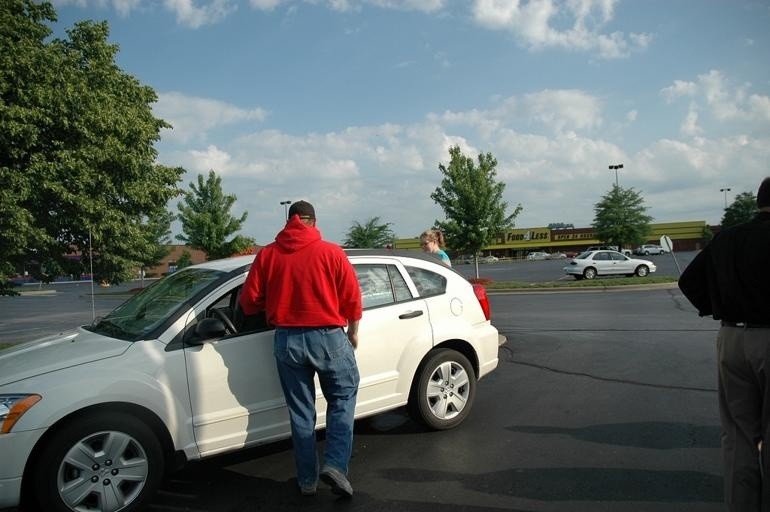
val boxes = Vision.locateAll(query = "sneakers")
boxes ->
[300,465,354,497]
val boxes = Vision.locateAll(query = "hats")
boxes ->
[288,200,315,221]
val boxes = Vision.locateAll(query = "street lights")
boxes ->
[608,164,623,188]
[281,200,292,221]
[721,188,730,209]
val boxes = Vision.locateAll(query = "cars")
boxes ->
[455,254,499,265]
[528,251,578,261]
[563,249,656,279]
[0,248,500,512]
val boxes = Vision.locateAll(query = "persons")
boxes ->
[673,177,770,512]
[239,200,363,498]
[419,227,453,269]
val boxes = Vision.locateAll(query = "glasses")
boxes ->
[421,242,429,246]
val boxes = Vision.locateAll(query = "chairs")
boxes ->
[358,271,424,308]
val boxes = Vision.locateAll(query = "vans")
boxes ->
[586,244,665,258]
